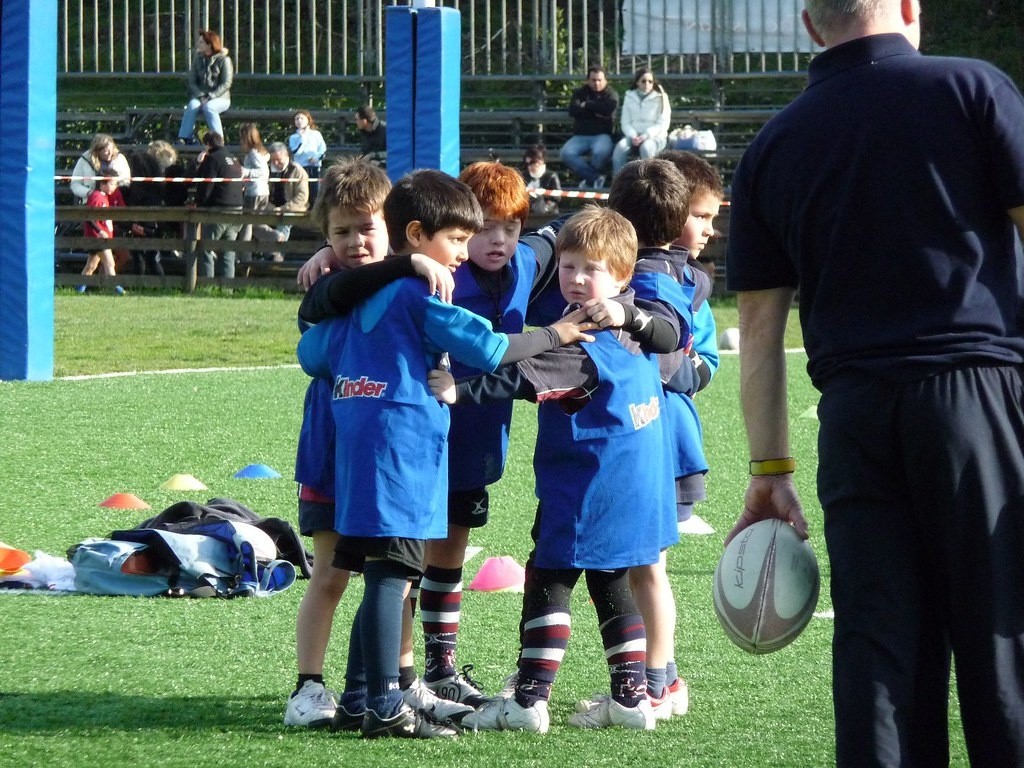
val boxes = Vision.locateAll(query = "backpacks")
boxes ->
[69,498,314,598]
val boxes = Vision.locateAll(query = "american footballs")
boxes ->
[711,517,822,656]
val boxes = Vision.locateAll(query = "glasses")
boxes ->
[638,79,654,84]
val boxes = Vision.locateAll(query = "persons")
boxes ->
[611,69,673,176]
[560,69,619,190]
[586,150,723,720]
[286,161,599,738]
[427,206,697,732]
[71,112,327,296]
[176,31,233,144]
[724,0,1024,768]
[522,147,560,213]
[355,107,385,168]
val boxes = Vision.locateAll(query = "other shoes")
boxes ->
[116,285,126,297]
[579,180,591,190]
[594,174,606,189]
[179,138,191,145]
[76,284,88,294]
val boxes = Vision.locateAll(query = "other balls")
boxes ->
[719,326,740,350]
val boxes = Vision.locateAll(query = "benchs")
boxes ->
[55,107,785,278]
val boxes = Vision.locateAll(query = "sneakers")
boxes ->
[495,669,522,698]
[461,694,549,733]
[401,673,475,726]
[329,705,366,733]
[569,691,656,731]
[575,685,671,720]
[360,700,457,739]
[284,679,339,728]
[421,664,491,710]
[668,677,688,716]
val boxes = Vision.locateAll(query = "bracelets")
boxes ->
[748,458,794,476]
